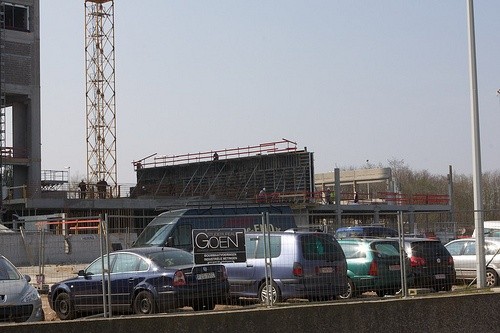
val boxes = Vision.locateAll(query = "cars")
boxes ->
[377,236,456,292]
[443,237,500,289]
[1,254,45,324]
[47,247,229,321]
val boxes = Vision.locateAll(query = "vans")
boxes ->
[224,232,349,304]
[132,204,298,252]
[471,221,500,245]
[334,225,400,239]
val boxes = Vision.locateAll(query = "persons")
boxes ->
[259,188,265,194]
[96,179,108,199]
[213,152,218,160]
[78,179,88,199]
[354,191,358,203]
[325,189,336,205]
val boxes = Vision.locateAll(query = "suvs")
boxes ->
[335,236,413,297]
